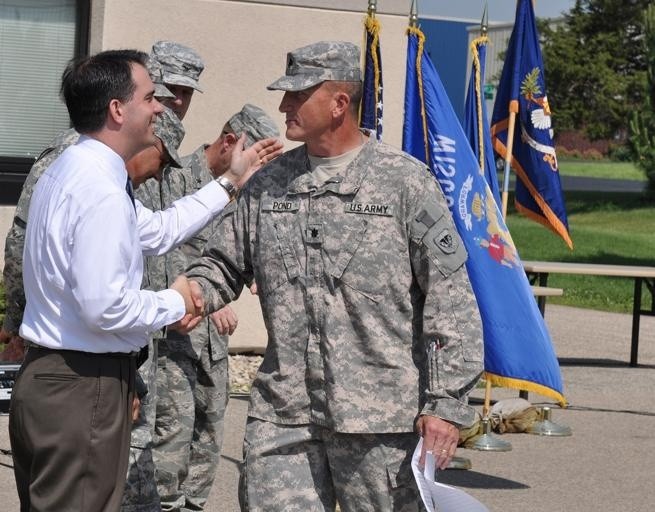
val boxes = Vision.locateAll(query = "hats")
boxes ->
[266,41,361,91]
[228,103,280,150]
[152,105,185,168]
[144,58,176,100]
[150,39,205,94]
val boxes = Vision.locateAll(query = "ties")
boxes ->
[126,175,137,217]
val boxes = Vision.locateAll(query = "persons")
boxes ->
[154,103,281,511]
[142,42,205,135]
[168,40,485,512]
[134,52,178,101]
[0,102,184,512]
[0,51,284,512]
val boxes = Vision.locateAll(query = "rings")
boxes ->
[259,159,264,164]
[441,448,448,453]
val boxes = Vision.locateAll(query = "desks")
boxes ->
[524,259,655,367]
[528,286,564,403]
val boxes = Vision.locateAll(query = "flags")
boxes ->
[402,28,567,409]
[459,33,503,214]
[490,1,575,251]
[355,13,385,143]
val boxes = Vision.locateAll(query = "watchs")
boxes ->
[214,176,236,197]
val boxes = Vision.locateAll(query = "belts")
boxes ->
[23,339,140,359]
